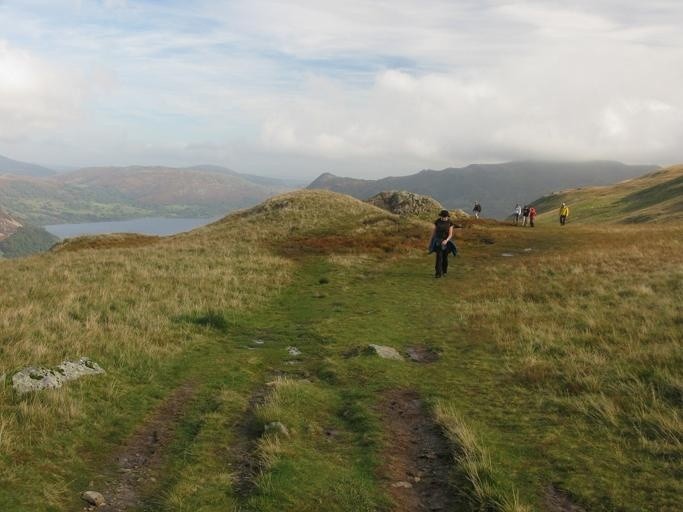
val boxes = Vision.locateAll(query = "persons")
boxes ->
[428,209,457,279]
[512,203,537,227]
[472,201,481,219]
[559,203,569,226]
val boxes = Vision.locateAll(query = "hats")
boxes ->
[438,210,450,217]
